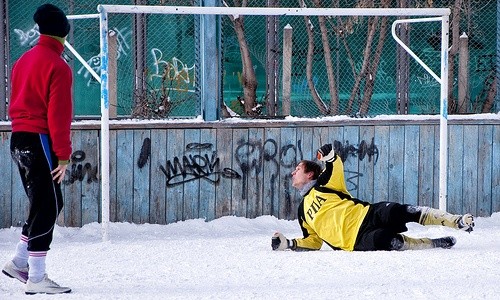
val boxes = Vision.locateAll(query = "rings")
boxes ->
[59,170,64,174]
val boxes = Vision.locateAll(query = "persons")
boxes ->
[271,144,475,251]
[1,4,74,295]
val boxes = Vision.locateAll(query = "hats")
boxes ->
[33,3,70,38]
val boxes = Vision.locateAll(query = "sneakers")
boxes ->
[24,273,72,296]
[2,260,28,285]
[431,235,458,249]
[456,213,475,233]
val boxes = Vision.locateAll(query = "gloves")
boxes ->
[271,232,291,252]
[317,143,336,162]
